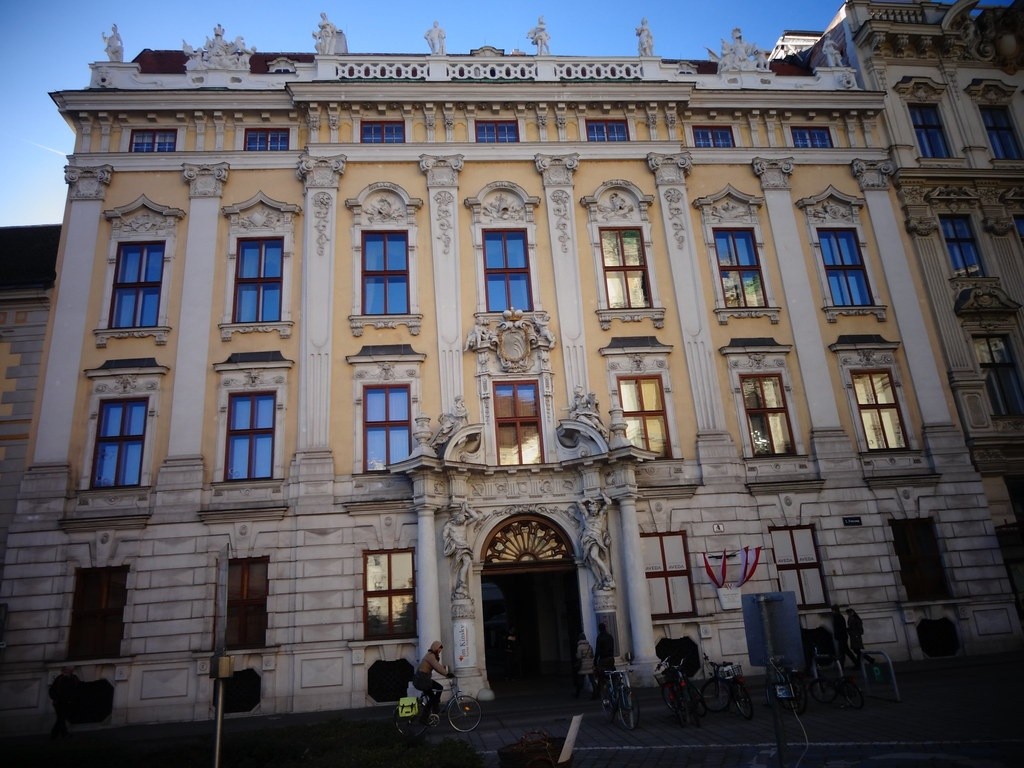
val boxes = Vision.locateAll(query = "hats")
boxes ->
[578,633,586,640]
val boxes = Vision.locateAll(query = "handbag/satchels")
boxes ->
[574,659,582,670]
[398,696,418,717]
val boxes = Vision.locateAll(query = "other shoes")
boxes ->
[852,663,861,671]
[419,717,431,727]
[433,709,445,714]
[504,682,511,688]
[511,682,517,687]
[589,695,597,700]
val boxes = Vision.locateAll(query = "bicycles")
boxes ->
[701,653,753,719]
[653,656,706,728]
[391,676,482,738]
[597,668,640,731]
[809,661,864,709]
[765,657,807,715]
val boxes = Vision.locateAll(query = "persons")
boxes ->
[533,313,557,350]
[635,18,654,57]
[576,493,616,592]
[447,396,471,433]
[823,33,843,68]
[594,623,615,694]
[442,503,479,601]
[831,604,858,671]
[718,28,773,73]
[318,13,336,56]
[845,608,876,670]
[101,24,123,62]
[181,24,256,71]
[568,385,606,434]
[414,640,454,728]
[425,22,445,55]
[462,316,486,351]
[577,634,599,700]
[526,17,550,55]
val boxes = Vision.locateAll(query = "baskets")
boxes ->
[718,665,743,680]
[654,672,674,689]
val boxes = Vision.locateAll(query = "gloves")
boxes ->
[593,660,599,667]
[447,673,455,678]
